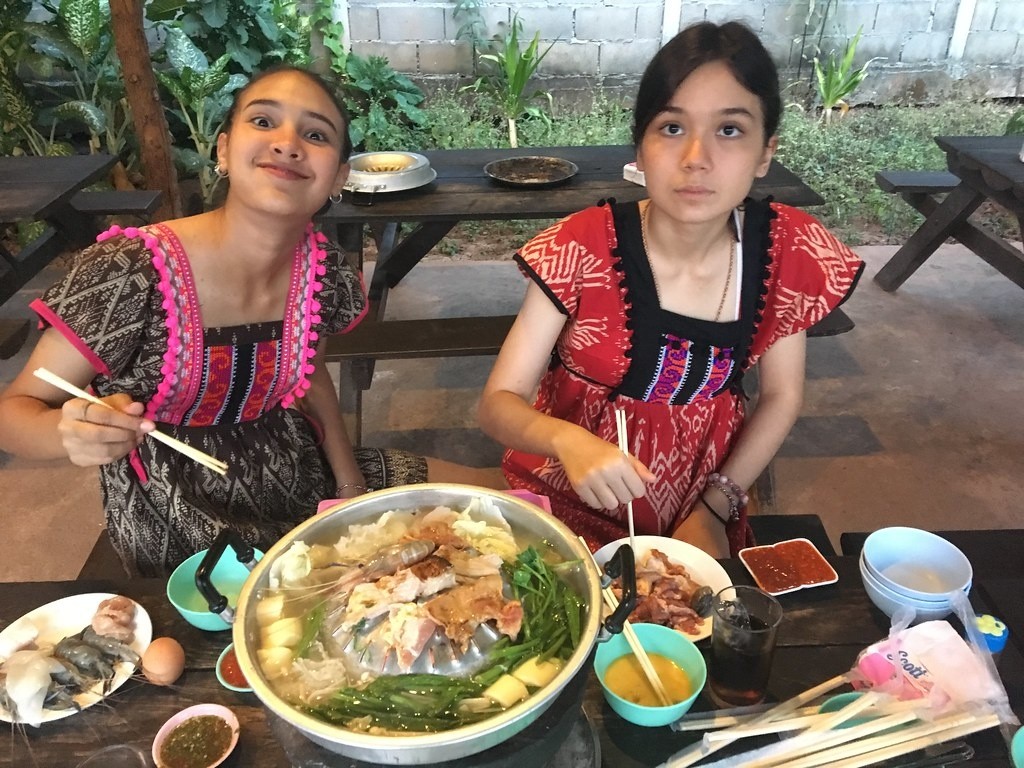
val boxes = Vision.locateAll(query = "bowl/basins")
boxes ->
[593,622,707,728]
[167,544,265,632]
[859,526,973,625]
[819,692,881,731]
[1009,725,1024,768]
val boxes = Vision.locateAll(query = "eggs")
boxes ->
[143,636,186,687]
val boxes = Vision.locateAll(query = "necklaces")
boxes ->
[641,200,735,321]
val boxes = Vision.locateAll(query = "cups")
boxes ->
[73,744,148,768]
[708,585,784,710]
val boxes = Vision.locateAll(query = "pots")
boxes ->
[194,483,636,765]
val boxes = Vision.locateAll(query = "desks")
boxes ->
[315,143,828,443]
[875,136,1024,290]
[2,555,1024,767]
[0,154,117,250]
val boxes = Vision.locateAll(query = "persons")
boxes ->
[478,22,867,563]
[0,67,512,579]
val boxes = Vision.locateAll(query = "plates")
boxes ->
[592,535,737,643]
[151,703,239,768]
[483,156,579,185]
[0,592,152,723]
[215,642,253,691]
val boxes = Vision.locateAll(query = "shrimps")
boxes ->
[81,627,182,691]
[0,646,78,721]
[54,636,116,695]
[339,538,435,593]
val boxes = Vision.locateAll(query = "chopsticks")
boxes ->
[33,368,229,475]
[667,674,1000,768]
[579,535,674,707]
[616,410,637,565]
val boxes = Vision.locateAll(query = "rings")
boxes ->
[83,403,93,420]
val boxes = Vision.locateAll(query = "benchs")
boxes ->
[70,188,164,215]
[74,514,840,578]
[324,298,856,360]
[875,170,960,192]
[0,318,31,359]
[839,530,1024,723]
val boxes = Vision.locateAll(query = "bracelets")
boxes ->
[335,484,374,498]
[701,496,728,528]
[709,483,739,522]
[707,472,749,507]
